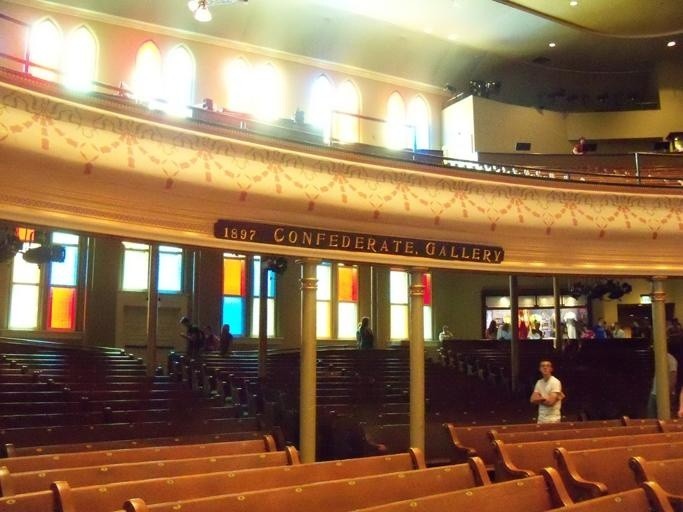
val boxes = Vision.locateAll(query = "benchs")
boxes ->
[444,153,683,197]
[0,335,682,511]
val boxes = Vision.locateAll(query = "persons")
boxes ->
[573,136,588,155]
[439,325,455,343]
[531,360,565,424]
[677,390,682,420]
[486,313,683,342]
[179,317,233,355]
[646,343,678,418]
[359,317,374,348]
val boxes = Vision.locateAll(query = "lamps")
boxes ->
[260,254,286,273]
[0,218,66,267]
[567,276,632,302]
[193,0,213,22]
[440,77,500,101]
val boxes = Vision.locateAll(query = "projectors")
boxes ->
[262,257,287,273]
